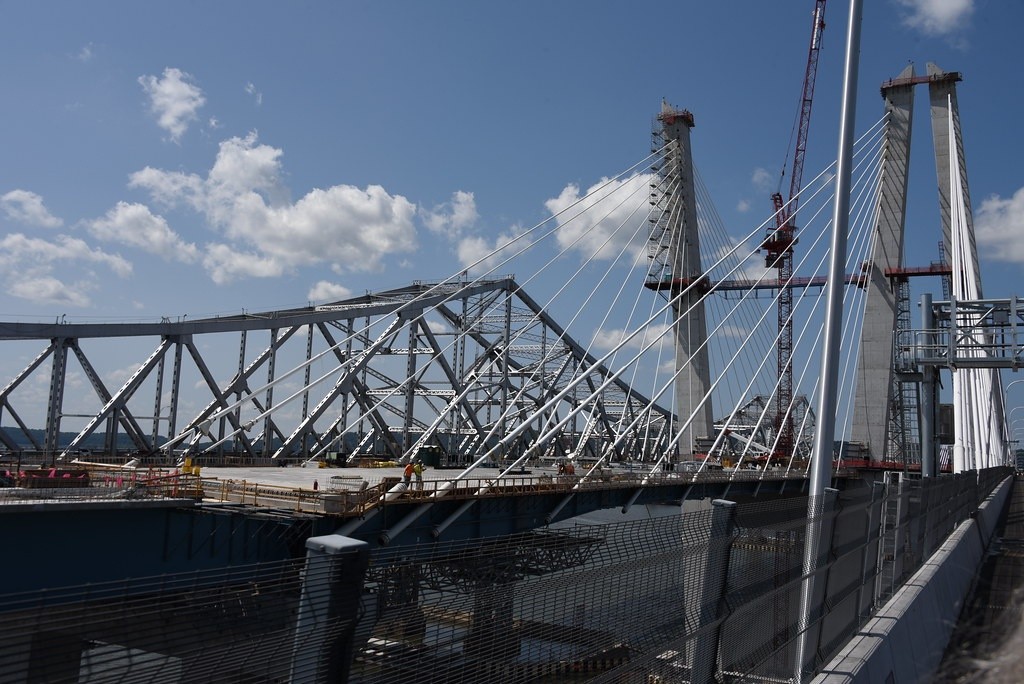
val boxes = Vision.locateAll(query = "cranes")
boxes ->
[754,0,828,470]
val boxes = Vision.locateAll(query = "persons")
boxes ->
[404,460,414,487]
[414,460,426,490]
